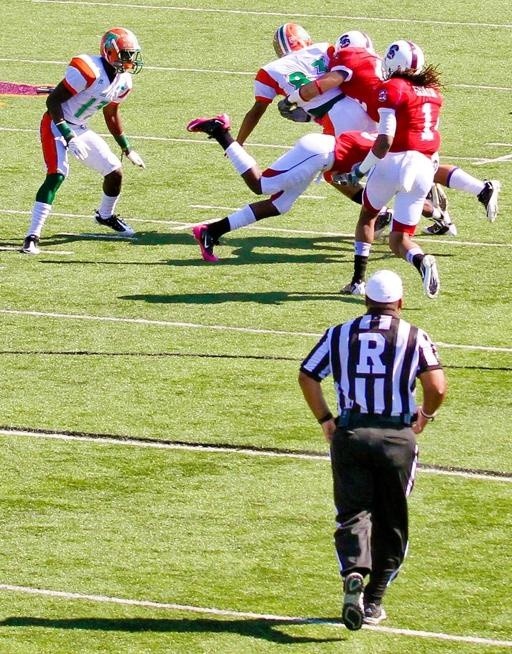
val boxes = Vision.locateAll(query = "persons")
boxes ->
[340,39,448,299]
[186,112,379,262]
[299,269,447,630]
[22,27,146,255]
[236,23,458,239]
[278,30,500,223]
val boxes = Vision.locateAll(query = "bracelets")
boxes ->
[319,411,333,425]
[420,408,436,422]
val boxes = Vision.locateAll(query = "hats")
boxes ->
[364,270,403,303]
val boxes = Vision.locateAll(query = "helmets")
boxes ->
[381,39,424,80]
[333,30,375,56]
[100,27,143,73]
[272,23,313,57]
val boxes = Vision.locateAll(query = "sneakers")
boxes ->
[422,223,457,235]
[94,209,134,235]
[373,207,393,239]
[341,571,364,630]
[187,114,230,135]
[24,234,40,253]
[192,224,218,261]
[477,179,499,222]
[419,253,440,297]
[340,280,365,295]
[363,602,386,624]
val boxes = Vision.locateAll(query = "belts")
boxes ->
[356,410,411,425]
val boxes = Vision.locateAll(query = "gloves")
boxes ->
[128,150,147,170]
[277,100,294,117]
[287,106,309,122]
[348,161,364,185]
[68,137,91,160]
